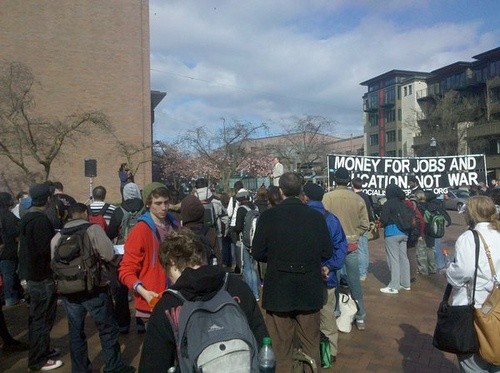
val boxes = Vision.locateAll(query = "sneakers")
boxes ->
[47,346,61,358]
[27,359,63,371]
[400,284,411,291]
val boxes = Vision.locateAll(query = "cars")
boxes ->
[444,188,471,212]
[479,187,500,206]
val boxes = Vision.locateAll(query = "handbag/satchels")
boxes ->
[473,281,500,367]
[432,301,479,354]
[292,346,318,373]
[336,292,359,333]
[319,337,333,369]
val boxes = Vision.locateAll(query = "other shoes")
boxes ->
[15,297,26,305]
[340,279,348,289]
[360,274,367,281]
[136,324,145,333]
[119,326,129,334]
[356,319,366,330]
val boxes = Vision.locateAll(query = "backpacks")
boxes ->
[117,205,147,245]
[159,271,265,373]
[85,203,109,232]
[419,204,446,238]
[389,197,416,233]
[50,221,100,297]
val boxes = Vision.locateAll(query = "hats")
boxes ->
[28,183,52,199]
[333,167,351,184]
[141,182,170,210]
[195,177,208,189]
[303,182,324,201]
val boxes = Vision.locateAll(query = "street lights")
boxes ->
[429,135,437,157]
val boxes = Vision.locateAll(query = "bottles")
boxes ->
[442,249,454,268]
[258,337,276,372]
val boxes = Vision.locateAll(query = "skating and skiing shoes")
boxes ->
[379,285,398,294]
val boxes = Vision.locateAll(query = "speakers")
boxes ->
[84,158,97,177]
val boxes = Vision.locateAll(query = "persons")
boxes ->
[271,157,284,187]
[118,163,134,203]
[0,168,500,372]
